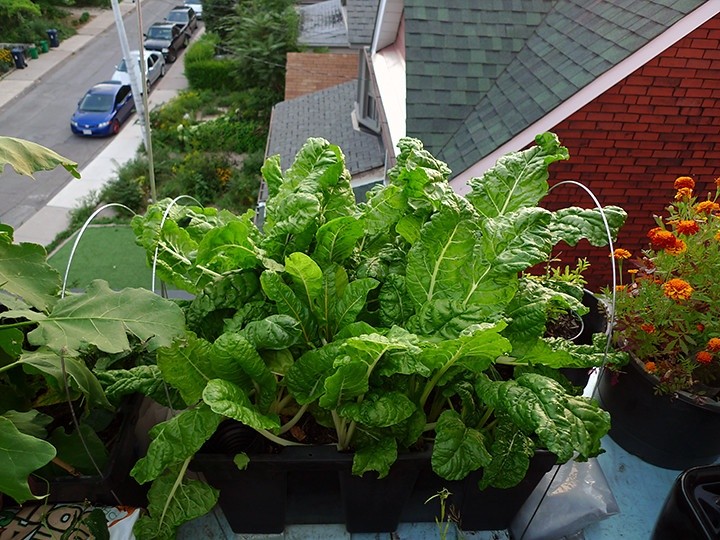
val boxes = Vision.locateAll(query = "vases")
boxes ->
[599,339,720,471]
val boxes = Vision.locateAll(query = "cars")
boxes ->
[69,81,134,138]
[143,21,191,64]
[111,49,166,96]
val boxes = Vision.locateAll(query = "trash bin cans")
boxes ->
[29,46,39,59]
[41,40,48,53]
[46,29,59,47]
[11,47,28,69]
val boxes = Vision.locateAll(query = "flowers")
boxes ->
[611,177,720,398]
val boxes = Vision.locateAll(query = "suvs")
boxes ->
[184,0,203,21]
[165,6,198,36]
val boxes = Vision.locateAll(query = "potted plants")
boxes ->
[0,223,143,505]
[189,137,629,534]
